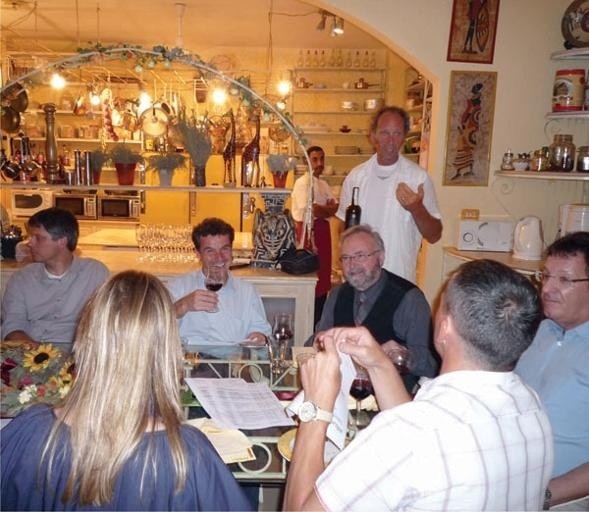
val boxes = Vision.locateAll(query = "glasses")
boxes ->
[338,250,380,264]
[534,270,589,290]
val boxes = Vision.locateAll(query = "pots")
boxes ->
[364,98,383,111]
[354,77,380,89]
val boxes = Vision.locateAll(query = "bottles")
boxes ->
[548,134,576,172]
[576,145,589,172]
[532,150,549,172]
[344,187,362,233]
[552,67,586,113]
[501,153,515,171]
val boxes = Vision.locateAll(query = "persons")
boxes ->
[336,105,445,288]
[0,207,112,354]
[165,216,273,350]
[299,224,442,401]
[514,231,589,512]
[449,82,484,180]
[281,258,554,511]
[289,145,339,334]
[462,1,484,54]
[1,268,253,512]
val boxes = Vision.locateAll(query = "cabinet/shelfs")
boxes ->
[1,183,297,231]
[402,64,433,171]
[286,66,388,187]
[0,81,140,173]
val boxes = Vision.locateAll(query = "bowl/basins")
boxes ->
[339,128,351,133]
[343,102,352,106]
[512,159,529,171]
[342,81,351,89]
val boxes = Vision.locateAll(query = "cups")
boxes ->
[273,312,293,361]
[266,334,288,375]
[135,223,202,263]
[204,262,227,313]
[384,344,415,381]
[349,367,373,426]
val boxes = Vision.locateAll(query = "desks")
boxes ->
[75,226,257,252]
[0,250,322,347]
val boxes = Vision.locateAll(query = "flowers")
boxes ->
[3,345,71,405]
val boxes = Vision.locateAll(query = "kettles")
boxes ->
[559,204,589,239]
[512,214,545,260]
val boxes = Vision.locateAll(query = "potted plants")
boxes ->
[82,149,108,186]
[148,148,188,187]
[109,144,145,185]
[170,108,218,187]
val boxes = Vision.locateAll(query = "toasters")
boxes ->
[454,216,514,253]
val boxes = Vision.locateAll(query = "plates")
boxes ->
[342,107,353,110]
[360,145,373,154]
[561,0,589,50]
[295,164,309,175]
[277,428,331,467]
[335,146,358,155]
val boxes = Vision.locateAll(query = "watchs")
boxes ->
[542,486,554,511]
[296,400,335,427]
